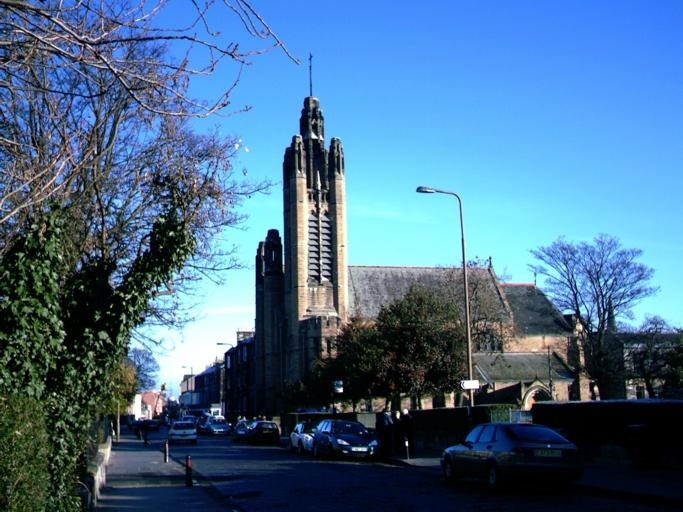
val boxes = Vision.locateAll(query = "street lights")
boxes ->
[181,366,192,415]
[415,185,475,410]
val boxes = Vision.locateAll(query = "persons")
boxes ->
[141,422,150,448]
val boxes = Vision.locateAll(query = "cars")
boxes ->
[440,422,582,493]
[167,415,381,459]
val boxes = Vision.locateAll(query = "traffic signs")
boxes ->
[460,380,479,390]
